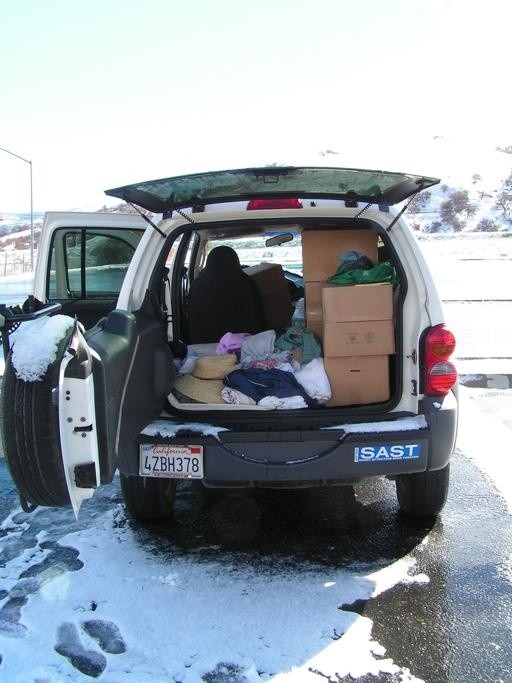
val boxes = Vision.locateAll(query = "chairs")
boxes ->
[187,248,266,344]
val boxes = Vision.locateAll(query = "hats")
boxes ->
[172,354,238,403]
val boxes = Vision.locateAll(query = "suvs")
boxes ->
[0,169,458,549]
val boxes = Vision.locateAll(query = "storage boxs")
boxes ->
[241,260,293,329]
[300,230,398,409]
